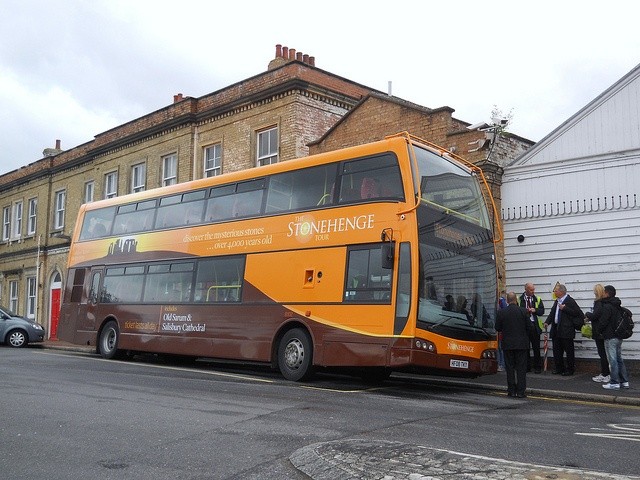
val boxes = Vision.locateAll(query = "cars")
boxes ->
[1,305,44,347]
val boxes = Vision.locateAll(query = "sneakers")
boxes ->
[622,382,629,388]
[602,383,620,390]
[592,375,610,383]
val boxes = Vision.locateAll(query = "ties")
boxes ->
[527,297,530,309]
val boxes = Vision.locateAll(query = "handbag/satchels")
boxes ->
[581,320,592,339]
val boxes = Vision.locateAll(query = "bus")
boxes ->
[55,130,502,381]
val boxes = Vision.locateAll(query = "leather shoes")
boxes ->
[535,369,540,374]
[561,370,573,376]
[552,370,562,374]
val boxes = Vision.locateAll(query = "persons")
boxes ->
[543,284,577,375]
[598,285,630,391]
[497,297,507,372]
[584,284,611,383]
[495,291,534,398]
[517,283,545,374]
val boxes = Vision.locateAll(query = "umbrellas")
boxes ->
[543,325,548,374]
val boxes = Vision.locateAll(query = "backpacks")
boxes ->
[614,303,634,339]
